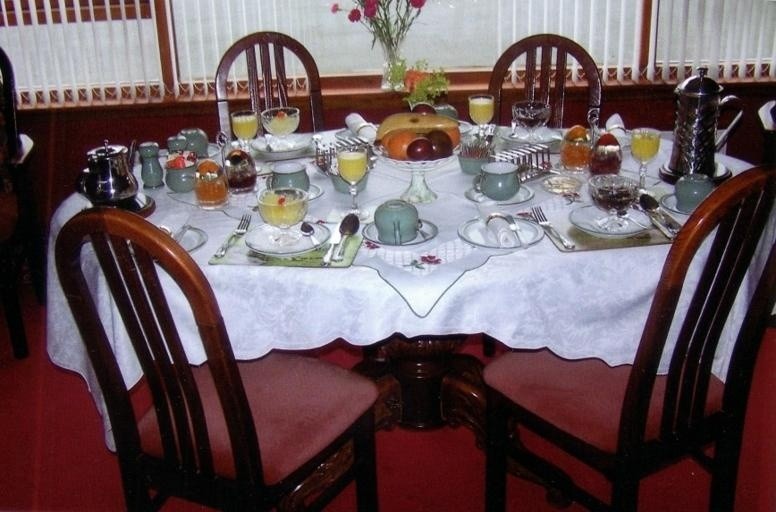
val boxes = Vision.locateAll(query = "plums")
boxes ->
[406,139,432,161]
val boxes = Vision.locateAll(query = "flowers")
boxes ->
[331,0,426,63]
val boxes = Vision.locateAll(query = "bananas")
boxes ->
[374,112,460,146]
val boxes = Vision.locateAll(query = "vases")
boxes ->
[381,61,406,88]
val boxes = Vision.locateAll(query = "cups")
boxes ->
[669,67,745,174]
[674,172,714,213]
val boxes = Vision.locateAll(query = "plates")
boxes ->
[167,129,691,258]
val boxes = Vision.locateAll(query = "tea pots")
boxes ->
[77,139,151,213]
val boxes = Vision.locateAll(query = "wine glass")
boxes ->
[231,93,661,246]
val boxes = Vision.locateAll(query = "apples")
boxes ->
[413,104,436,117]
[425,130,452,161]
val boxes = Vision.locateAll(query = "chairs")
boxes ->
[758,100,776,164]
[489,35,602,129]
[481,165,776,512]
[217,33,324,141]
[54,207,380,512]
[0,47,45,359]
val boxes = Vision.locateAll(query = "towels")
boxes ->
[606,114,627,143]
[345,112,376,140]
[480,202,517,248]
[155,212,190,243]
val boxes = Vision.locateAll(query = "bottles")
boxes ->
[139,141,163,190]
